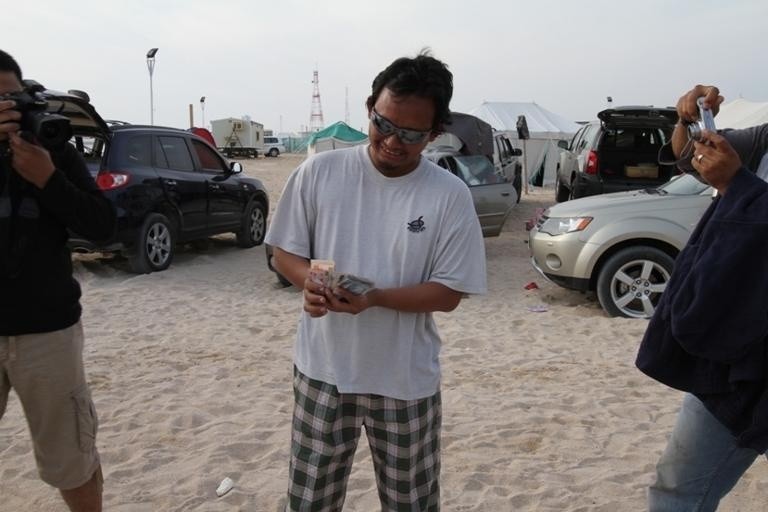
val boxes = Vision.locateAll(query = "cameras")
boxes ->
[687,95,718,142]
[0,79,73,148]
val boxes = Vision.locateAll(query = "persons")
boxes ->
[255,46,490,512]
[629,82,767,512]
[0,46,108,512]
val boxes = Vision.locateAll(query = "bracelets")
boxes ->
[678,116,692,128]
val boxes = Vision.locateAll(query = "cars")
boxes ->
[40,88,269,274]
[410,127,523,238]
[263,136,286,157]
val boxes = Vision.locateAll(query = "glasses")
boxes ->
[370,106,434,145]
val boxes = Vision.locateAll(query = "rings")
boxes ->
[697,155,704,163]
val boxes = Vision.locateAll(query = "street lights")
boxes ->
[200,95,205,128]
[145,48,159,124]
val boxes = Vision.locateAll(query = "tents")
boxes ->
[422,113,502,184]
[467,98,583,193]
[307,119,370,159]
